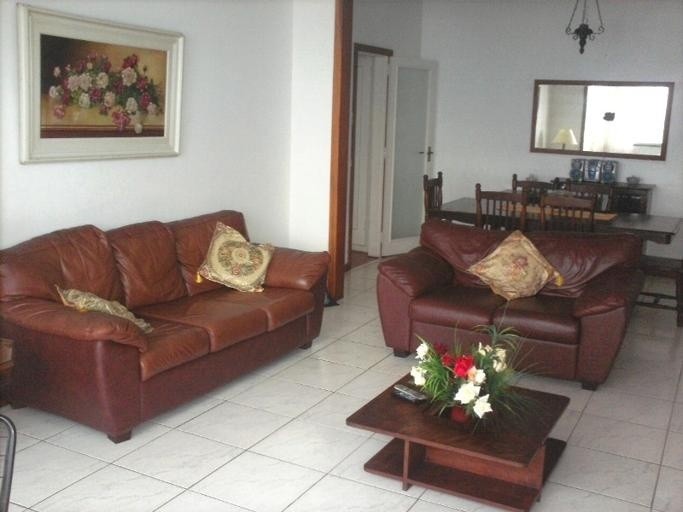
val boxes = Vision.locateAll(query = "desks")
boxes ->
[439,195,683,308]
[553,177,656,252]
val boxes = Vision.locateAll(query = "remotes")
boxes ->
[391,384,427,405]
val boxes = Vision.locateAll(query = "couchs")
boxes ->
[0,209,331,443]
[376,220,643,391]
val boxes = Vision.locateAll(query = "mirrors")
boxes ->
[529,79,674,161]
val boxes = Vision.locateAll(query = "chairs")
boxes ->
[423,173,614,232]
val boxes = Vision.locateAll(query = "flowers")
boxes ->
[410,301,548,438]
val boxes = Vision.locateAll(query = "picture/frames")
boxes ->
[17,4,185,164]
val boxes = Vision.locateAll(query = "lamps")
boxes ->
[551,128,578,149]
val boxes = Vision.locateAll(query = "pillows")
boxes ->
[57,287,154,333]
[196,220,275,292]
[466,229,558,298]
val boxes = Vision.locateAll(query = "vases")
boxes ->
[450,404,471,423]
